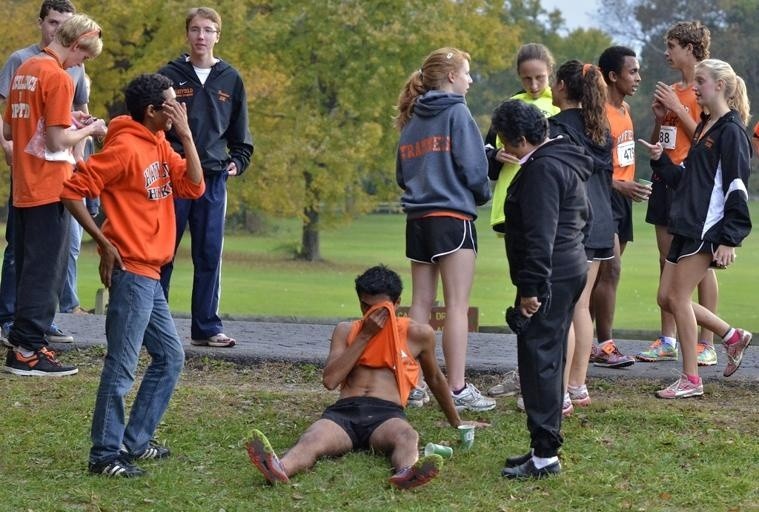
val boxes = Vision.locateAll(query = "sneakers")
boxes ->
[450,383,496,412]
[593,327,753,373]
[388,454,444,489]
[655,375,703,399]
[487,368,591,416]
[191,332,236,348]
[501,450,561,480]
[119,442,171,460]
[407,385,430,408]
[88,456,147,478]
[4,348,79,377]
[245,428,290,485]
[48,331,75,344]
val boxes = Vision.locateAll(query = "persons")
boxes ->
[0,0,109,377]
[244,264,443,492]
[60,73,207,480]
[483,20,759,480]
[389,46,498,413]
[156,5,254,349]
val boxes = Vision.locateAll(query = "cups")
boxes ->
[637,176,653,202]
[457,423,476,449]
[424,441,453,460]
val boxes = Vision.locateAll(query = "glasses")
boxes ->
[189,27,218,37]
[71,29,102,51]
